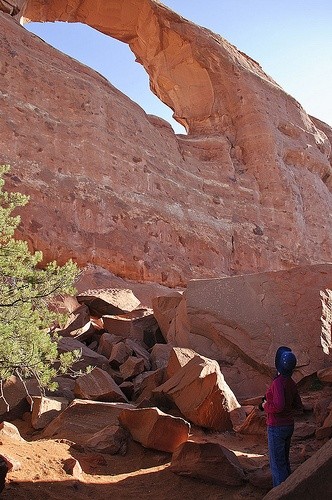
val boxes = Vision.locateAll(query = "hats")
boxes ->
[275,346,297,378]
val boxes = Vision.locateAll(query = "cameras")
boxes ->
[259,397,266,412]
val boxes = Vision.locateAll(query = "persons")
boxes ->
[262,346,303,488]
